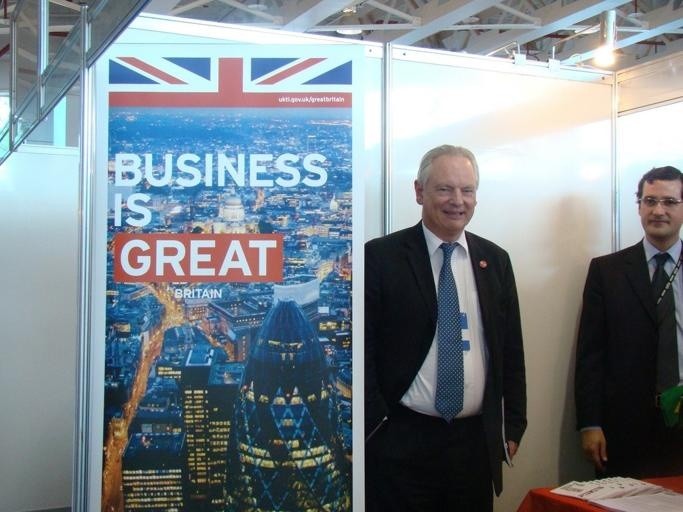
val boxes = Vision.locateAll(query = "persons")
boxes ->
[365,144,528,511]
[574,166,683,482]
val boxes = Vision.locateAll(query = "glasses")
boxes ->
[637,197,683,209]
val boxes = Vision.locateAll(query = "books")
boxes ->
[499,394,514,468]
[549,476,683,512]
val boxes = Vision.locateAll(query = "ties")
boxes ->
[435,243,464,425]
[652,254,679,392]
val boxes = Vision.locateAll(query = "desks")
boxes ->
[528,476,682,512]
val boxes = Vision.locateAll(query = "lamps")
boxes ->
[336,16,361,35]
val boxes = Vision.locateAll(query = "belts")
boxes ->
[655,393,663,408]
